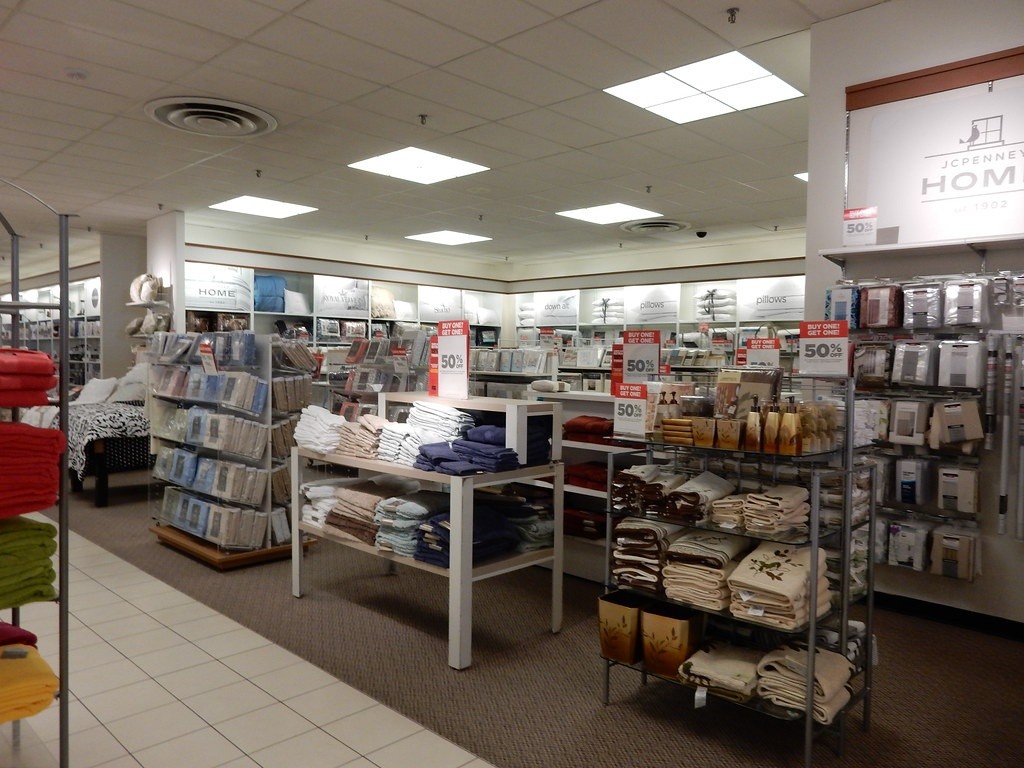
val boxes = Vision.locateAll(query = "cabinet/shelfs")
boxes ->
[0,182,880,768]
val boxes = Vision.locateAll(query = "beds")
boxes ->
[19,362,165,508]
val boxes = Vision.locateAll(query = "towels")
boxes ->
[292,401,554,477]
[609,463,811,533]
[298,472,555,569]
[0,341,69,729]
[607,515,833,631]
[560,414,648,541]
[677,618,882,727]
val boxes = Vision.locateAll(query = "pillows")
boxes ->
[518,311,535,319]
[126,317,144,334]
[520,303,534,310]
[77,376,118,403]
[140,308,158,333]
[698,298,735,308]
[697,314,735,322]
[284,289,311,314]
[520,319,534,325]
[130,273,152,301]
[592,317,623,323]
[593,306,624,313]
[698,305,737,314]
[157,313,170,332]
[393,300,416,320]
[141,279,158,303]
[592,298,623,306]
[694,291,734,299]
[476,308,500,326]
[592,312,624,318]
